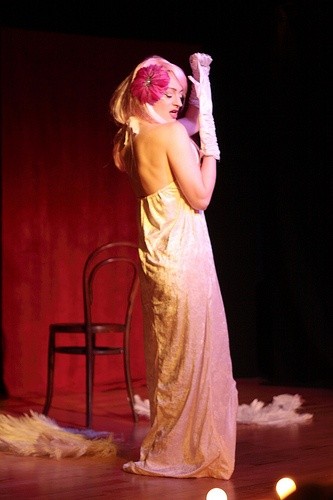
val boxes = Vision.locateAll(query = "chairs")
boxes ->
[39,240,139,432]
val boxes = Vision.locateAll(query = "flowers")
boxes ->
[129,64,170,106]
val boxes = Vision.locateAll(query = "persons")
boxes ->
[109,52,238,480]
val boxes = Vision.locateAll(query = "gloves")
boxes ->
[189,65,220,161]
[187,51,213,108]
